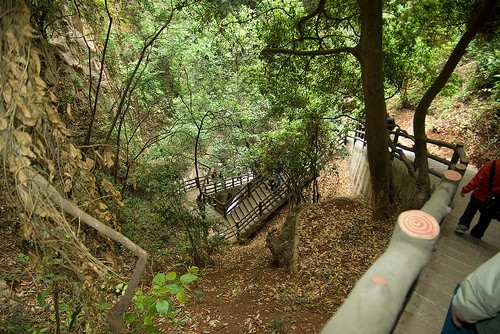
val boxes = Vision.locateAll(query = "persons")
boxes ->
[387,112,396,130]
[444,251,500,334]
[267,172,283,193]
[454,155,500,240]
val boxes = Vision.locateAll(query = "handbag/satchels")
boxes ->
[479,193,500,220]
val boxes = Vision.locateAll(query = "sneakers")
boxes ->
[455,224,468,235]
